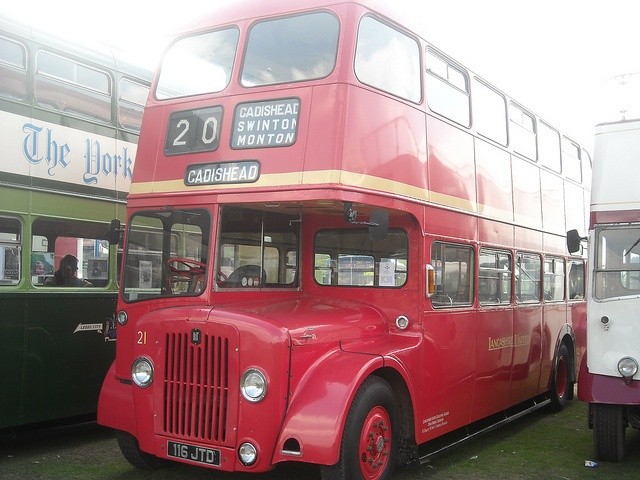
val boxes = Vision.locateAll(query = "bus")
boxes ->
[567,105,640,462]
[97,1,591,476]
[0,12,156,441]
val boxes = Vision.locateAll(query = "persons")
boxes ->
[43,255,95,287]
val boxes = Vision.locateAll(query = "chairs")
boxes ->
[225,266,266,287]
[431,295,452,307]
